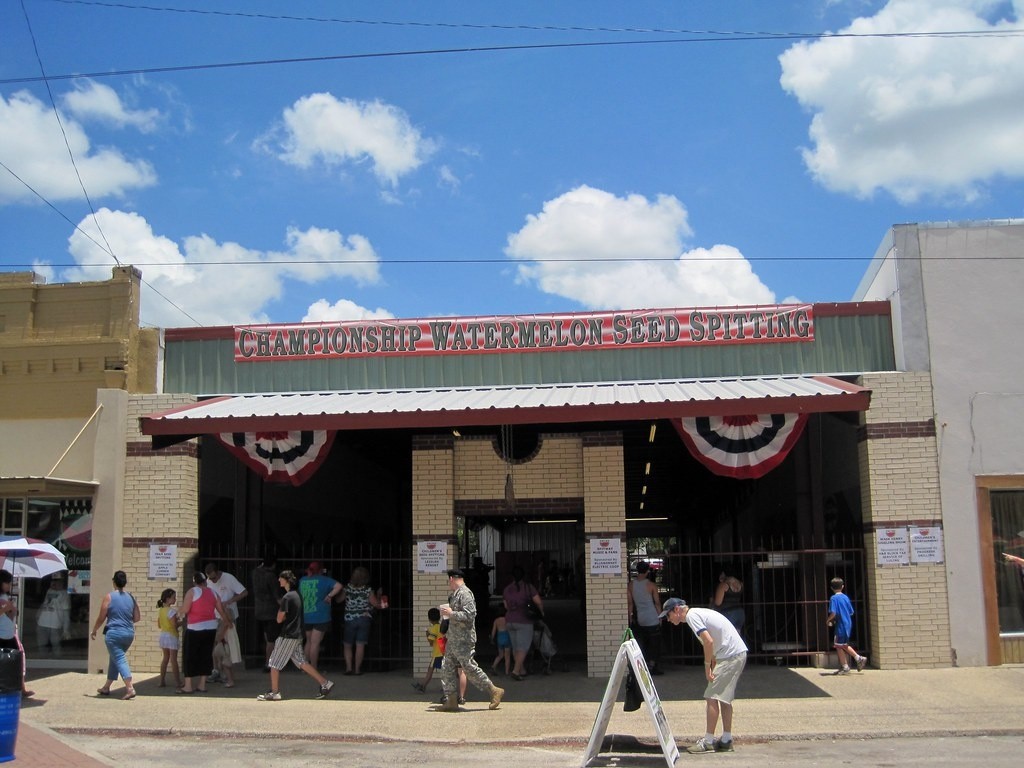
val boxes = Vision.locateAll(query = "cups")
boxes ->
[439,603,451,620]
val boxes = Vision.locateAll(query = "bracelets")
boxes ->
[327,594,332,599]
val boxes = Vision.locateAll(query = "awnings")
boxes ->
[138,375,874,450]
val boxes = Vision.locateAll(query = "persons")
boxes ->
[156,589,186,688]
[297,562,342,674]
[90,571,140,700]
[714,566,745,635]
[204,563,248,688]
[334,567,389,675]
[826,577,867,674]
[0,569,34,698]
[250,554,287,673]
[434,569,504,712]
[409,564,544,705]
[627,561,665,675]
[1002,552,1024,569]
[658,598,749,754]
[34,577,71,659]
[256,569,334,701]
[176,572,233,694]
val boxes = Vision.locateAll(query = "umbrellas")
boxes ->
[0,535,69,602]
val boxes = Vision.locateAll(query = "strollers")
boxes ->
[528,614,559,676]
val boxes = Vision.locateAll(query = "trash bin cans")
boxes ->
[0,648,23,764]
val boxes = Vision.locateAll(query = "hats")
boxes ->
[658,598,686,619]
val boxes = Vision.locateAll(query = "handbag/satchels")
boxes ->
[437,636,447,656]
[524,582,542,621]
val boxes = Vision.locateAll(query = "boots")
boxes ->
[486,685,504,710]
[435,692,458,711]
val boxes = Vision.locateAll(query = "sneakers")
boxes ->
[316,679,334,699]
[257,689,281,700]
[834,665,850,675]
[713,736,733,752]
[856,656,867,672]
[687,738,715,754]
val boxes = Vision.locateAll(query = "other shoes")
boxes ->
[207,671,221,682]
[649,666,664,675]
[457,697,465,705]
[439,695,448,702]
[22,690,35,698]
[411,682,425,694]
[489,667,497,675]
[343,670,361,676]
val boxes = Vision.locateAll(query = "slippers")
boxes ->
[97,688,109,695]
[122,693,136,699]
[521,673,526,676]
[511,672,523,681]
[195,687,207,692]
[174,685,194,694]
